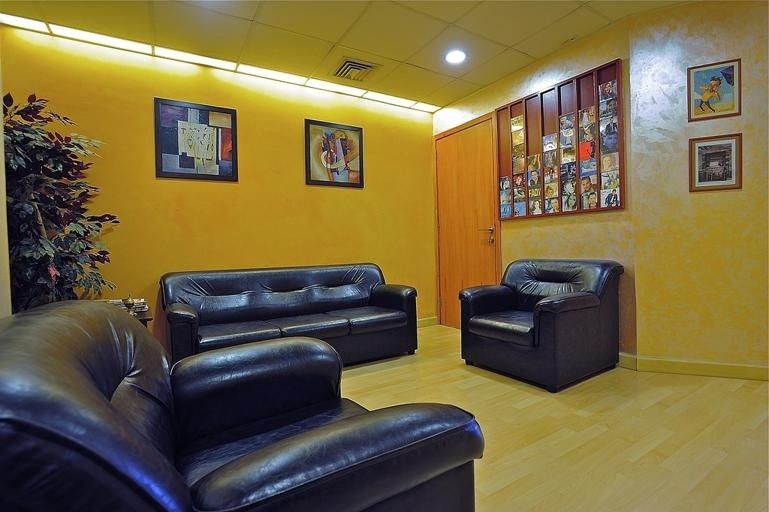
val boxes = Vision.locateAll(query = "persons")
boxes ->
[698,77,723,114]
[498,79,621,218]
[325,130,352,184]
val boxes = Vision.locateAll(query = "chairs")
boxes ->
[460,256,623,389]
[0,300,482,510]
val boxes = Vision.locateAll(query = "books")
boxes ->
[109,298,147,313]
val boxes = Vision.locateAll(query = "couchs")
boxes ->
[153,261,416,367]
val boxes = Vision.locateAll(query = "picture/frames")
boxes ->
[152,96,240,182]
[686,58,742,192]
[304,117,365,190]
